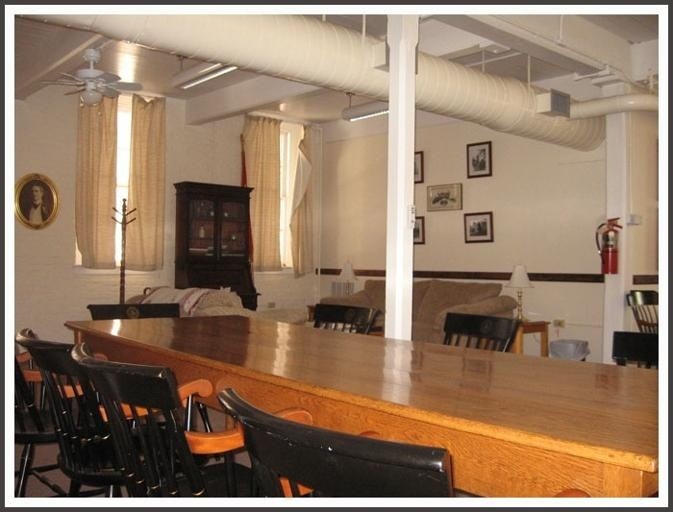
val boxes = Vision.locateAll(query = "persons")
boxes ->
[25,184,51,222]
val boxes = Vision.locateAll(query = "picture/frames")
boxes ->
[413,216,425,245]
[462,212,493,244]
[466,141,491,178]
[14,172,59,230]
[427,183,462,210]
[413,151,424,183]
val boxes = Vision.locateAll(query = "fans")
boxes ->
[37,51,141,97]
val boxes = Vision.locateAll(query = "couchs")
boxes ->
[320,279,517,345]
[125,288,322,318]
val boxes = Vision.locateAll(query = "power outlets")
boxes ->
[266,303,276,310]
[550,320,565,330]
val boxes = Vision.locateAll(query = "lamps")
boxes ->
[171,54,237,95]
[335,259,358,297]
[341,91,387,123]
[505,265,535,312]
[78,89,101,105]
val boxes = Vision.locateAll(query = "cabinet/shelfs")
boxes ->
[172,180,261,311]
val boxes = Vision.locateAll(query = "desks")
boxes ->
[509,320,550,357]
[63,308,658,498]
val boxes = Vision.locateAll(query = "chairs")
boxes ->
[14,356,67,498]
[441,311,520,352]
[311,305,373,333]
[15,327,215,497]
[610,329,658,371]
[214,384,456,498]
[86,303,179,319]
[71,341,316,498]
[625,289,658,334]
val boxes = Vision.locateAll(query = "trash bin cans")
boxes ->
[548,338,588,362]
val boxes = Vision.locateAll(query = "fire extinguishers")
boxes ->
[595,217,623,275]
